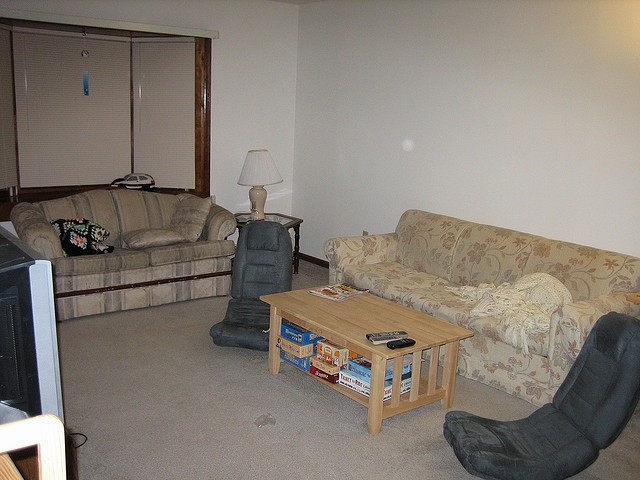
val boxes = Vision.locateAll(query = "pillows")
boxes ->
[123,228,183,249]
[169,196,214,242]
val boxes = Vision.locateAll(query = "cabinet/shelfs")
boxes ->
[0,413,66,479]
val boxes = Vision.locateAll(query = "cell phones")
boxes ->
[387,339,416,350]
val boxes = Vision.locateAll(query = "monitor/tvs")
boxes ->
[1,225,65,425]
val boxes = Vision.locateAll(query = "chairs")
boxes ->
[209,222,293,352]
[442,311,639,480]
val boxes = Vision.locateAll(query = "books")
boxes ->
[307,282,370,301]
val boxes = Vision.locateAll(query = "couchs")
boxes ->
[323,210,640,410]
[10,189,238,324]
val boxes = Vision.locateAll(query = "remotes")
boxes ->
[365,331,408,340]
[369,335,408,346]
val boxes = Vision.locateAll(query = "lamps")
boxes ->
[237,149,284,221]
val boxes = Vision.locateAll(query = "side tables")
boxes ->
[230,210,303,275]
